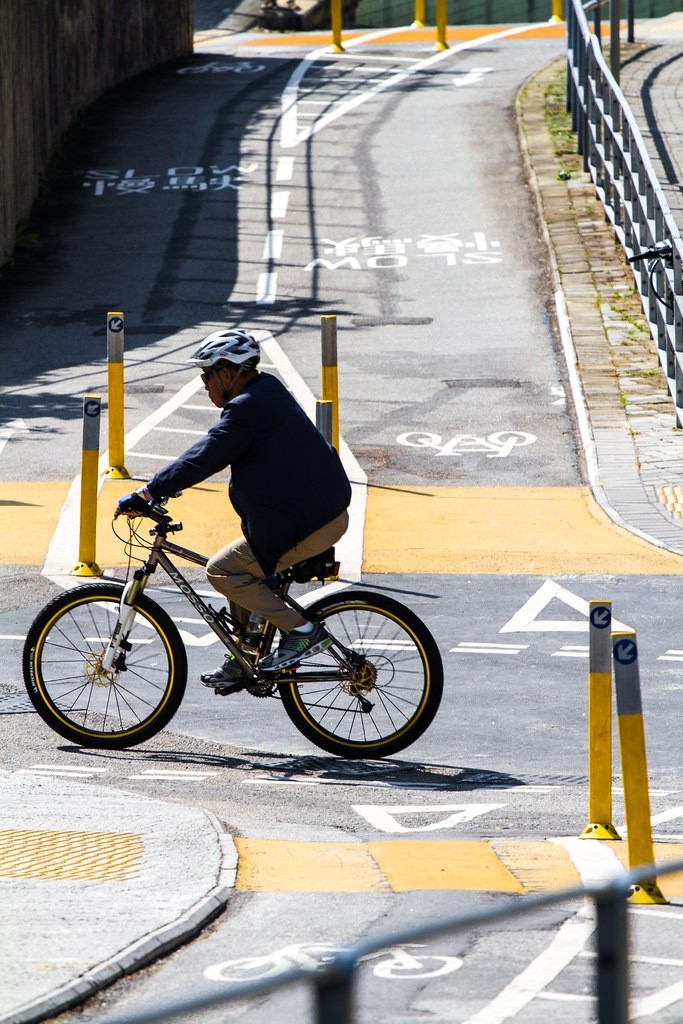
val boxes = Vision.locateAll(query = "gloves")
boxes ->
[116,486,154,517]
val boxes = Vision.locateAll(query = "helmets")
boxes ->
[186,330,260,370]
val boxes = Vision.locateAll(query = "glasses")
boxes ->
[201,366,231,384]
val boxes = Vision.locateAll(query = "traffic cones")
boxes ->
[106,311,131,480]
[321,317,340,454]
[70,395,105,576]
[610,630,669,906]
[580,599,620,841]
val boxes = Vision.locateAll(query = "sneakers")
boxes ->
[201,641,273,688]
[258,621,333,671]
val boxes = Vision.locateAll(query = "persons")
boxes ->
[116,330,352,687]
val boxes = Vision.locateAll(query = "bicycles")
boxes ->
[20,493,448,760]
